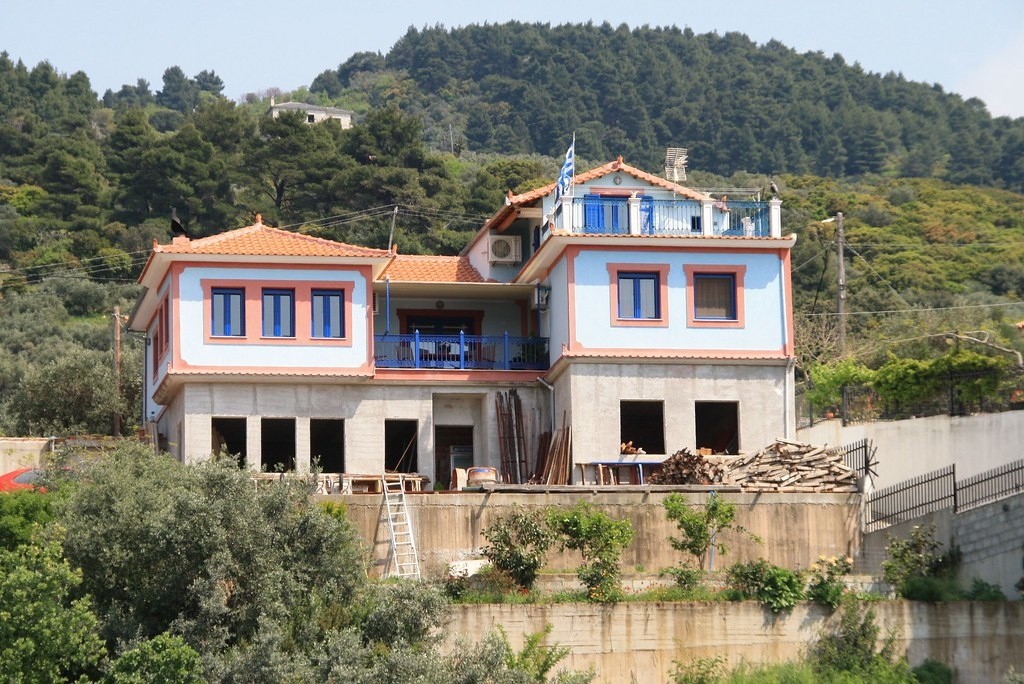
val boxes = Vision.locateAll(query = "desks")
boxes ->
[420,353,472,369]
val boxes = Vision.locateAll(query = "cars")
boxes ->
[0,466,86,494]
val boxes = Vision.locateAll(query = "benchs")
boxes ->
[338,473,432,495]
[575,461,660,486]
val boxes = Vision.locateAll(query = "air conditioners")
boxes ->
[372,291,380,316]
[488,235,522,267]
[530,286,549,310]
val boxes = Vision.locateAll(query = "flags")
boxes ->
[558,142,574,188]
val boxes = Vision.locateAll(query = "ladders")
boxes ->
[371,471,432,586]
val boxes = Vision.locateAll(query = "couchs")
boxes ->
[467,344,496,369]
[397,347,413,366]
